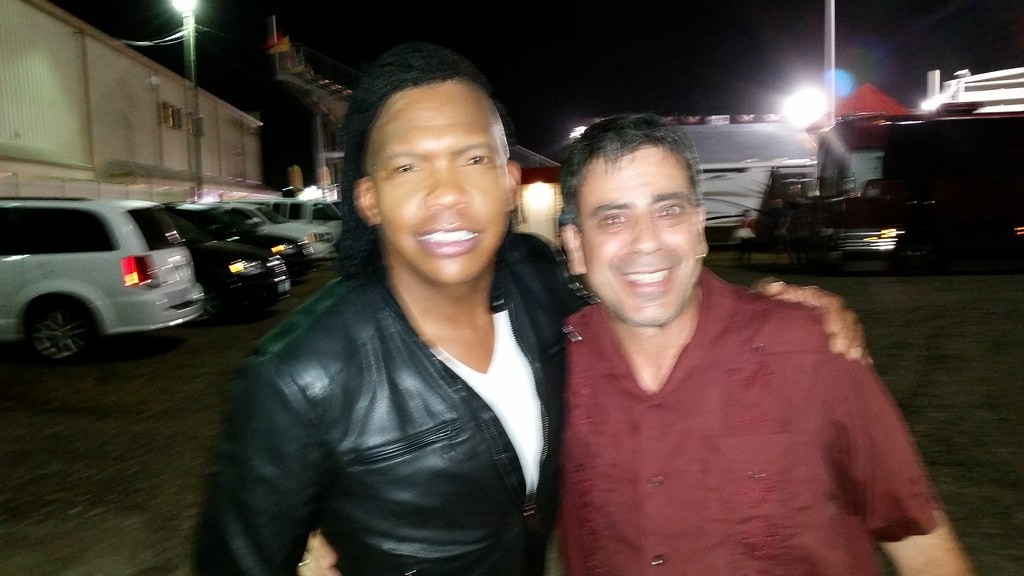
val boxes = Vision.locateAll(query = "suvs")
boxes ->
[0,197,207,370]
[218,197,345,259]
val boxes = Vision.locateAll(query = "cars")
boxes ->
[164,209,293,323]
[162,202,335,255]
[181,204,320,278]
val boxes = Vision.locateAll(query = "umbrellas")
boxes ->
[807,84,922,134]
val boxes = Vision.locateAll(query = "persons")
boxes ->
[298,116,980,576]
[189,49,873,576]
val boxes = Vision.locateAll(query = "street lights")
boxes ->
[172,0,205,204]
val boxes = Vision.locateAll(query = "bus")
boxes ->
[809,105,1024,273]
